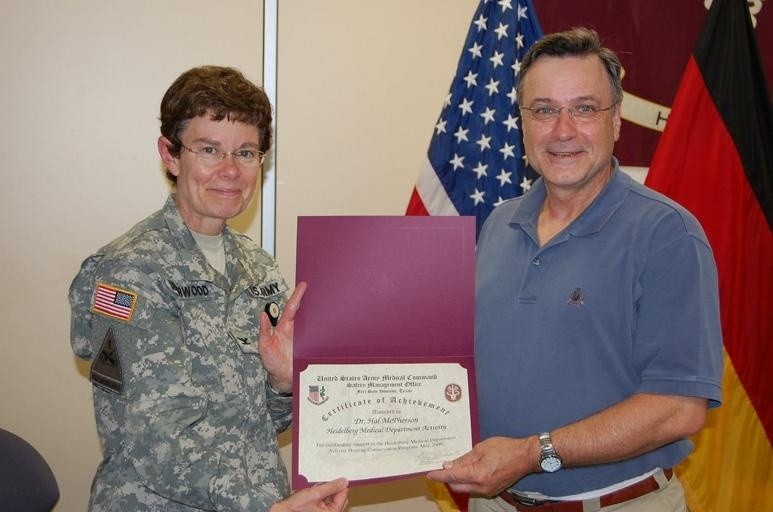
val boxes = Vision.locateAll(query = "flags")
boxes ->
[403,1,553,511]
[640,2,773,512]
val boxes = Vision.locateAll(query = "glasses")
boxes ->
[518,100,618,119]
[177,143,267,169]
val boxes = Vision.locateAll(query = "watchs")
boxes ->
[538,431,563,476]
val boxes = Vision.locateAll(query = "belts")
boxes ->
[497,464,676,512]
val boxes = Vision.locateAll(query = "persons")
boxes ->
[67,65,351,511]
[423,29,725,511]
